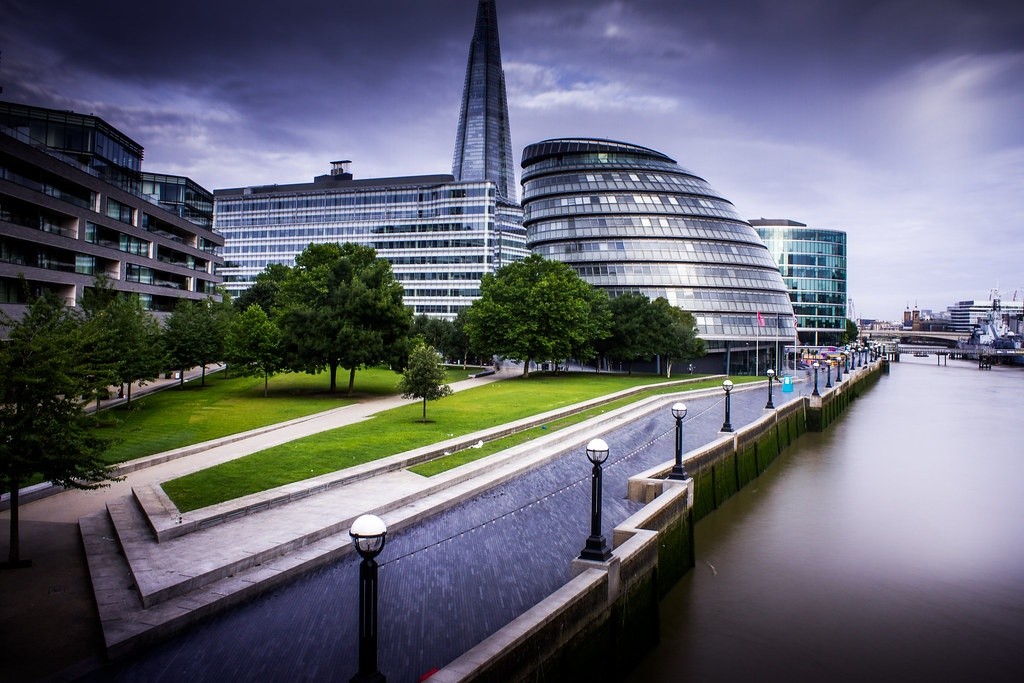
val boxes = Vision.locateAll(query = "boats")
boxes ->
[914,351,929,357]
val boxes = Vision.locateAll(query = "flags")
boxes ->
[794,313,798,330]
[757,309,766,326]
[777,312,781,329]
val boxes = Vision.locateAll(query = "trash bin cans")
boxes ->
[542,363,549,371]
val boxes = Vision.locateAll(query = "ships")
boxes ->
[949,299,1024,367]
